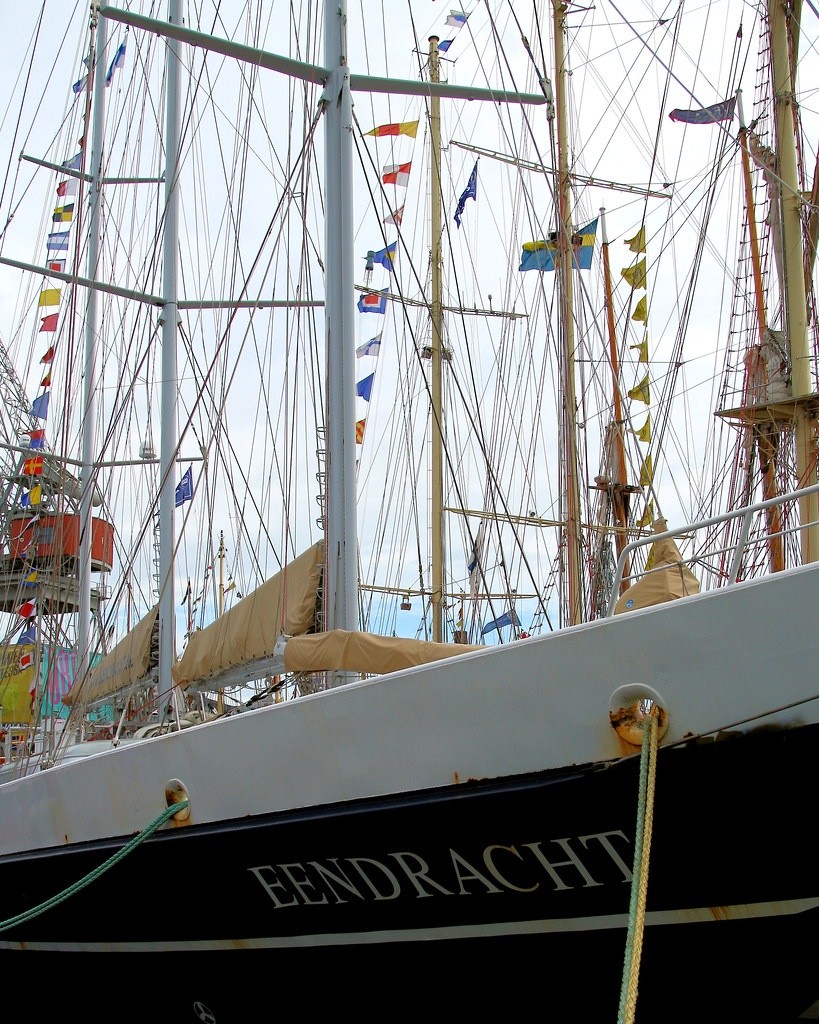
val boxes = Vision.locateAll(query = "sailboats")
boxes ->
[3,0,819,1024]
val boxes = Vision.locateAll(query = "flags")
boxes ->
[673,97,739,123]
[0,8,651,727]
